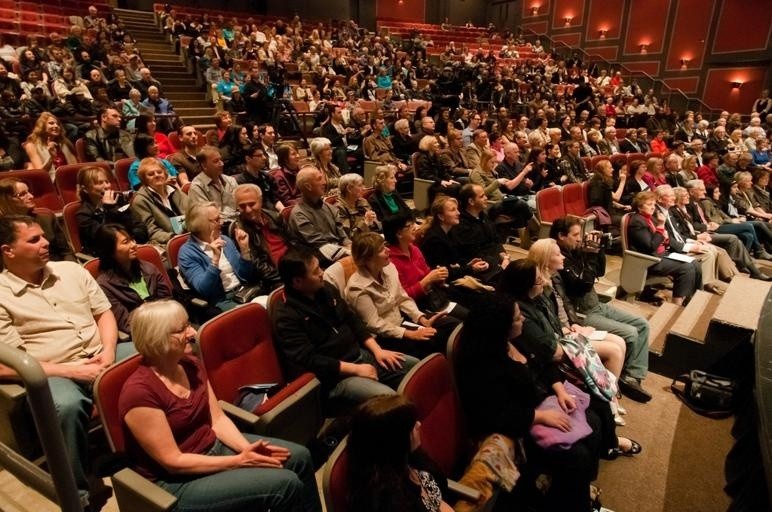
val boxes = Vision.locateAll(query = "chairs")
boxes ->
[74,138,91,163]
[98,4,111,12]
[533,186,584,250]
[22,24,42,33]
[238,60,251,74]
[309,84,317,93]
[393,100,407,109]
[19,2,42,12]
[100,13,112,22]
[358,186,377,200]
[280,204,296,223]
[226,217,240,239]
[36,207,55,222]
[321,75,335,90]
[2,21,18,30]
[41,5,63,15]
[150,1,330,51]
[55,161,124,205]
[82,10,95,18]
[555,85,566,98]
[336,74,346,90]
[206,128,218,147]
[42,14,63,26]
[1,9,18,22]
[168,130,204,154]
[284,63,298,77]
[291,84,299,101]
[0,362,43,460]
[65,17,84,25]
[578,157,593,172]
[627,154,644,164]
[359,102,379,124]
[265,286,291,311]
[316,428,478,511]
[591,157,610,173]
[327,254,362,288]
[646,152,661,159]
[22,33,46,47]
[408,99,426,123]
[299,74,314,86]
[262,63,267,74]
[583,178,619,238]
[444,320,469,346]
[561,182,600,243]
[377,16,544,67]
[62,200,96,263]
[117,158,138,191]
[79,244,173,297]
[617,210,662,298]
[615,128,625,142]
[333,48,346,55]
[408,150,442,206]
[398,354,557,512]
[163,231,222,322]
[1,1,19,11]
[46,24,67,37]
[1,32,20,47]
[292,102,315,135]
[566,84,575,97]
[358,137,386,185]
[320,193,339,205]
[0,170,61,214]
[19,12,42,24]
[416,79,427,89]
[62,7,81,17]
[195,302,323,428]
[95,338,203,512]
[65,26,86,37]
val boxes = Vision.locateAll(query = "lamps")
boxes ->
[600,30,607,35]
[638,45,648,50]
[564,18,571,22]
[532,7,538,11]
[731,82,743,89]
[680,60,688,65]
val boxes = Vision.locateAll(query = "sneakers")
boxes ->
[749,272,772,282]
[754,250,772,260]
[619,375,652,401]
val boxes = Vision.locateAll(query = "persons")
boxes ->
[627,191,700,305]
[453,290,603,512]
[528,236,628,427]
[91,223,171,335]
[737,153,755,175]
[674,186,739,280]
[496,142,540,211]
[369,166,409,223]
[417,130,488,196]
[644,157,665,191]
[0,214,136,512]
[751,138,772,172]
[0,5,200,185]
[272,242,421,409]
[157,5,771,159]
[718,179,771,245]
[344,233,448,361]
[752,167,772,212]
[588,160,631,228]
[545,142,566,187]
[488,194,533,230]
[698,152,719,188]
[500,258,642,460]
[655,185,716,289]
[177,202,258,310]
[289,168,351,261]
[417,195,488,278]
[188,145,236,213]
[230,183,298,284]
[470,153,540,250]
[630,160,651,196]
[382,209,467,328]
[237,142,283,213]
[702,183,772,260]
[549,216,653,402]
[611,154,639,208]
[306,138,340,181]
[115,299,312,512]
[560,139,590,186]
[131,157,191,245]
[0,178,75,261]
[733,172,771,222]
[333,173,382,240]
[718,152,737,186]
[679,155,697,182]
[686,180,771,281]
[454,182,511,276]
[274,145,301,205]
[664,153,683,187]
[74,166,144,250]
[348,393,455,512]
[528,148,548,190]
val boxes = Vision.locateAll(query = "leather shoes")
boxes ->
[622,439,643,454]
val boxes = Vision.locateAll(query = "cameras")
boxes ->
[583,232,614,249]
[113,190,135,206]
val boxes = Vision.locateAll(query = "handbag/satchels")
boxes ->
[418,284,449,311]
[233,282,260,303]
[230,382,280,414]
[671,368,738,418]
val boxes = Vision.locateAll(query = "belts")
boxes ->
[225,283,243,295]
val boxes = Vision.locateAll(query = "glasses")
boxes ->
[534,279,545,286]
